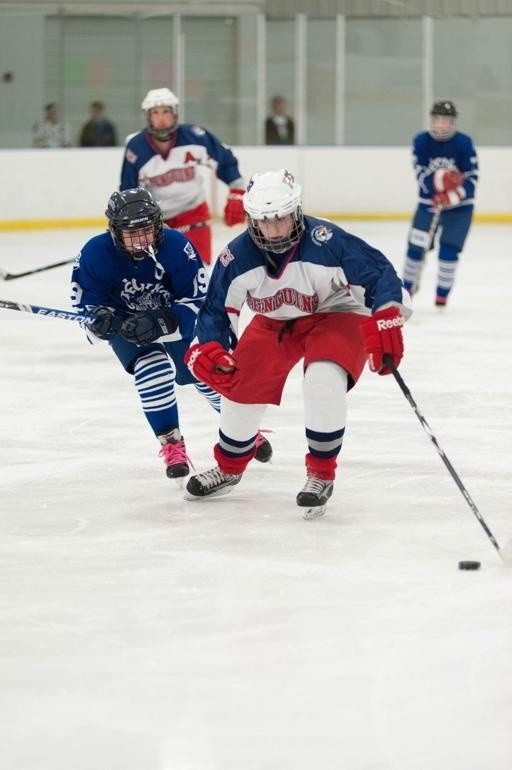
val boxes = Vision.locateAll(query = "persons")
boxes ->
[401,98,480,310]
[70,184,274,479]
[114,85,247,266]
[179,168,414,506]
[265,94,294,146]
[31,102,74,148]
[79,100,117,148]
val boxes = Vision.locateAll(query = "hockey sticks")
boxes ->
[383,356,511,567]
[2,259,73,280]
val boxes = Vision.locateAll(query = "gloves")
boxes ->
[224,188,247,226]
[121,304,179,345]
[85,305,117,338]
[434,168,463,191]
[184,341,242,398]
[433,184,466,210]
[359,306,404,377]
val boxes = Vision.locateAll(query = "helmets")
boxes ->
[243,168,305,255]
[141,88,180,142]
[430,100,457,140]
[105,187,166,262]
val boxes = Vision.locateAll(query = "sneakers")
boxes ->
[255,433,272,462]
[186,466,242,496]
[157,426,189,478]
[297,477,333,507]
